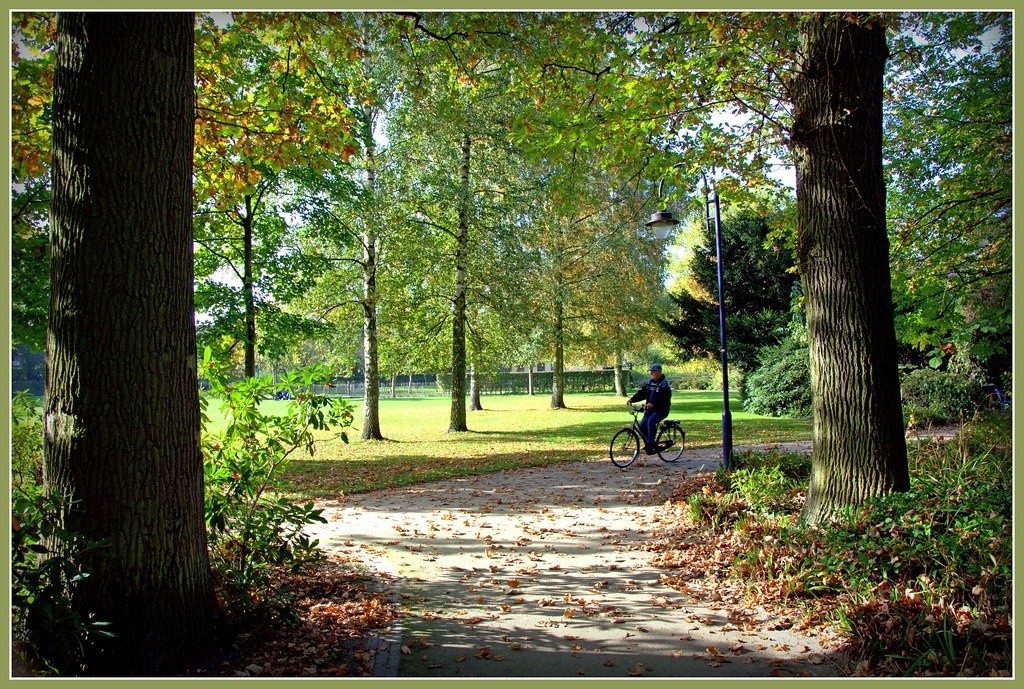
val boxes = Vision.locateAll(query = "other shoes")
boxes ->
[643,441,655,450]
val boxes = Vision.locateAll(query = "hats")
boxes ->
[647,364,662,372]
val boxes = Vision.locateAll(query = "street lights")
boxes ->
[645,161,734,470]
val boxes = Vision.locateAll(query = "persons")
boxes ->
[626,363,672,450]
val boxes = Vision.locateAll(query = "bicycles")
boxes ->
[610,401,685,468]
